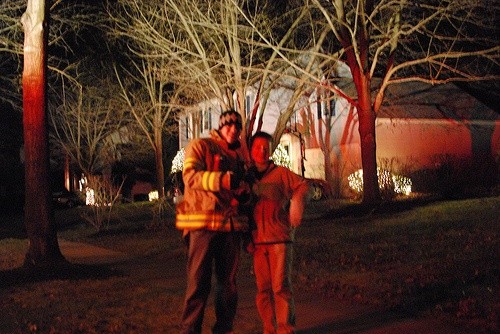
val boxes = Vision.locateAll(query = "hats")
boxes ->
[218,110,242,131]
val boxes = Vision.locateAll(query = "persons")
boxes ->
[175,110,250,334]
[242,131,308,334]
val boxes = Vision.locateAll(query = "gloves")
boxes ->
[223,171,239,191]
